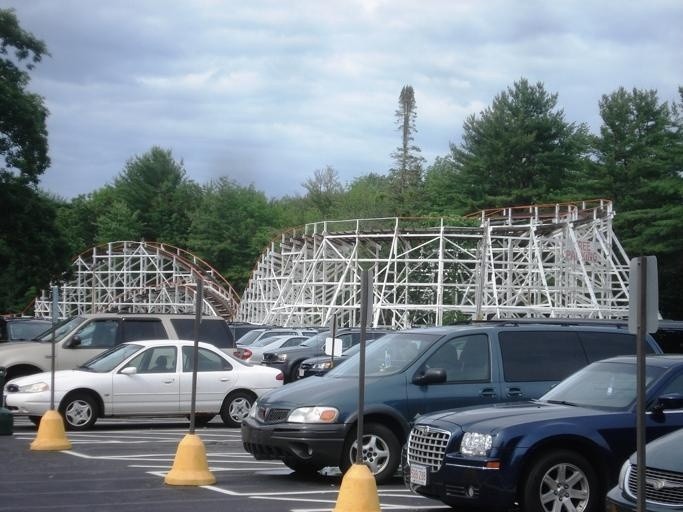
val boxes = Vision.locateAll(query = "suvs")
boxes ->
[0,313,235,389]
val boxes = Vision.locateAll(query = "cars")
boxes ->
[229,319,395,383]
[1,318,52,341]
[241,318,665,483]
[1,339,285,427]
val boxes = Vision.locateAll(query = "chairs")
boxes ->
[150,356,169,370]
[503,330,602,381]
[425,336,489,380]
